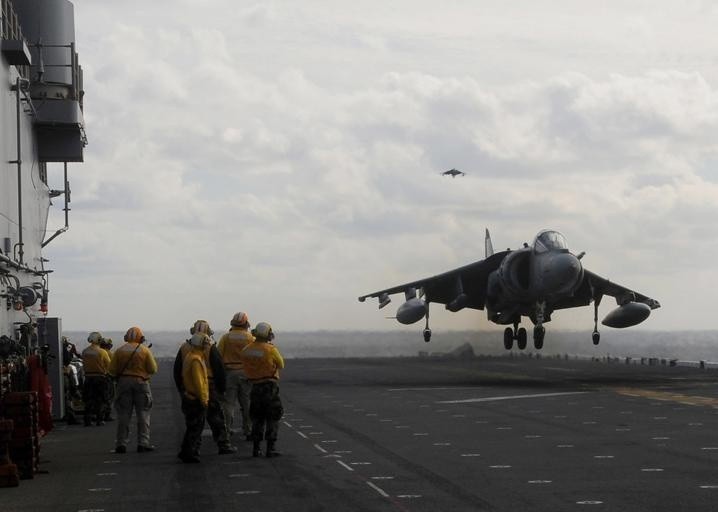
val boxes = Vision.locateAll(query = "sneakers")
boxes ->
[115,428,283,463]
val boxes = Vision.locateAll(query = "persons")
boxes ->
[99,336,117,423]
[174,331,211,464]
[79,331,114,427]
[214,312,257,443]
[172,318,238,455]
[239,320,285,458]
[107,325,158,456]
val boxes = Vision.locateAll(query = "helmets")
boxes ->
[190,319,215,348]
[231,312,248,325]
[255,321,272,338]
[126,326,142,342]
[88,331,114,346]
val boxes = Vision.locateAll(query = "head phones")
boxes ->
[251,328,275,341]
[123,334,146,343]
[230,319,250,329]
[188,326,214,337]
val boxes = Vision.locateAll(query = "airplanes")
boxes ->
[442,169,464,178]
[359,228,660,348]
